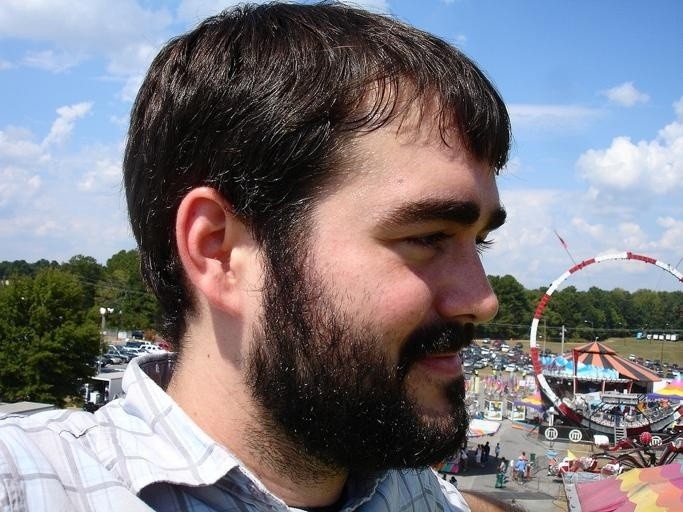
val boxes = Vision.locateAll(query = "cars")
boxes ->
[76,329,170,406]
[459,335,558,379]
[628,353,682,379]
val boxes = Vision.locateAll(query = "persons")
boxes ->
[448,475,457,488]
[441,473,447,480]
[474,442,528,484]
[0,0,510,511]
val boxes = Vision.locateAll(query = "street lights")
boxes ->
[618,322,625,345]
[584,320,593,342]
[98,307,106,372]
